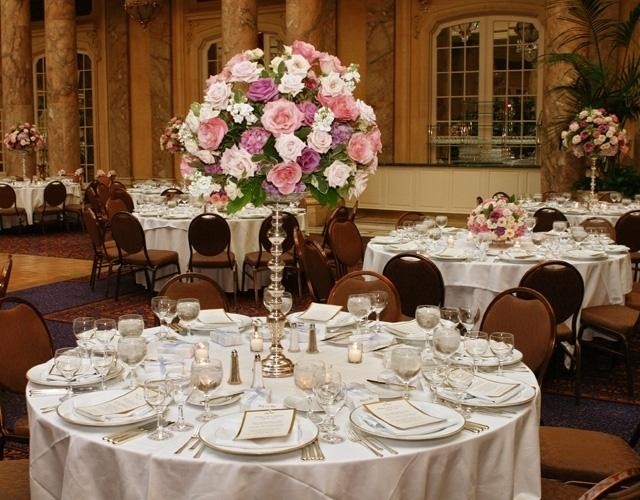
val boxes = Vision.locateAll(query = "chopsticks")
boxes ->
[301,443,327,461]
[30,389,101,397]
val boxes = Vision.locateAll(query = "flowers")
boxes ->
[180,39,383,215]
[3,121,44,154]
[159,115,185,155]
[560,107,629,159]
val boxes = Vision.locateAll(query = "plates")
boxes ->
[381,319,458,342]
[428,247,475,260]
[373,235,409,244]
[605,244,630,253]
[349,398,466,441]
[563,249,609,260]
[198,411,316,457]
[26,356,123,387]
[498,251,546,263]
[236,210,273,219]
[286,392,323,412]
[185,386,240,407]
[57,389,173,428]
[384,242,421,252]
[163,214,191,220]
[287,306,356,327]
[141,211,163,216]
[185,311,252,332]
[327,330,365,345]
[434,365,524,407]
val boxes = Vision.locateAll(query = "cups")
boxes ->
[277,292,293,316]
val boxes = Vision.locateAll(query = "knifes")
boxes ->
[103,420,174,444]
[200,392,245,402]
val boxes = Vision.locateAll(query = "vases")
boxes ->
[588,158,598,208]
[22,154,31,182]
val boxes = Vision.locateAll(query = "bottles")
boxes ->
[227,349,242,385]
[307,323,319,354]
[288,323,301,352]
[251,354,265,388]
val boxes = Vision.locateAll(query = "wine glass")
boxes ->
[518,218,615,253]
[294,290,515,444]
[55,297,223,444]
[516,189,640,214]
[397,210,449,247]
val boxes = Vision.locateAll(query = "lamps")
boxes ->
[124,1,161,33]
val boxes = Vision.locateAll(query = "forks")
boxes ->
[348,431,399,456]
